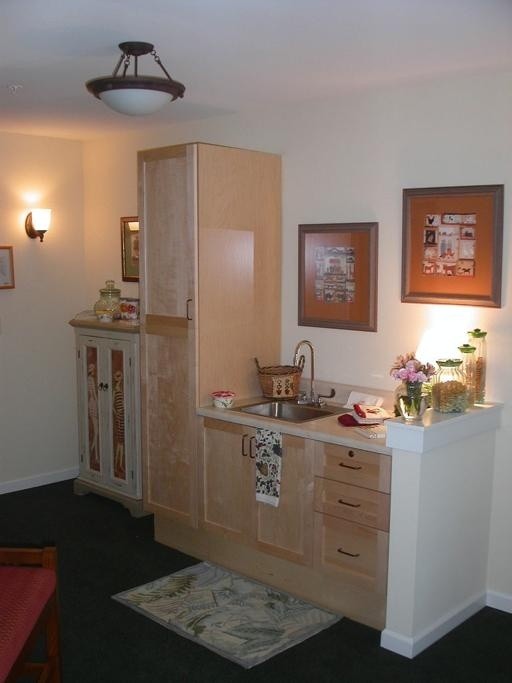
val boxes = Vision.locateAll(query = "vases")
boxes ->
[396,389,430,422]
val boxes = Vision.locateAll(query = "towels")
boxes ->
[252,427,283,507]
[336,413,380,426]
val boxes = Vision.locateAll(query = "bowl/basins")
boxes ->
[207,390,237,410]
[98,312,114,322]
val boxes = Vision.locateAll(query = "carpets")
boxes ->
[109,559,349,671]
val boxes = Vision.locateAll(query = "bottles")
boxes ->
[458,328,487,412]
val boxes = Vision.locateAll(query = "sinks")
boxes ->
[240,401,332,420]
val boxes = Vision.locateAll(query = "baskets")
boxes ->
[254,355,305,398]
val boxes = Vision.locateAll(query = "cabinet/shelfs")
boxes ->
[72,321,145,502]
[196,414,319,570]
[137,143,282,520]
[313,439,393,586]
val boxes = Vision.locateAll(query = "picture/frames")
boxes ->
[297,223,380,332]
[0,246,17,291]
[400,183,502,307]
[118,215,143,283]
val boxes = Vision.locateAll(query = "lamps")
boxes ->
[84,40,188,116]
[24,206,52,243]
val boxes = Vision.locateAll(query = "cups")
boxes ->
[120,296,139,320]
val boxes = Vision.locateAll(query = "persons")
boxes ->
[87,362,99,463]
[112,369,124,473]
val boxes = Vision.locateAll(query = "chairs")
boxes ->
[0,544,62,683]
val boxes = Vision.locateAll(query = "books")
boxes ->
[352,403,390,425]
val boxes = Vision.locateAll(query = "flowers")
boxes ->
[392,353,440,388]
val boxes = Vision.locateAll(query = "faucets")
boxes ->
[293,341,314,404]
